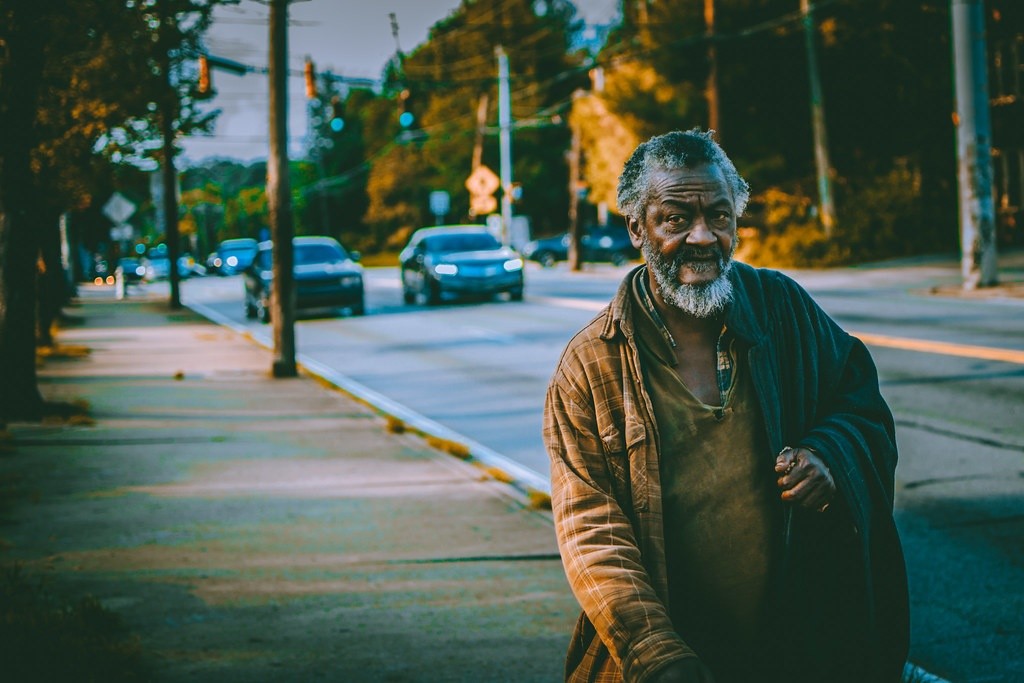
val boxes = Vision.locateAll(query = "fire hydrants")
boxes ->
[114,263,129,301]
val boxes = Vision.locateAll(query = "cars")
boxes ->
[521,225,642,267]
[94,235,259,283]
[244,234,367,323]
[398,223,524,306]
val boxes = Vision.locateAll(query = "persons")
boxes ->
[542,126,910,683]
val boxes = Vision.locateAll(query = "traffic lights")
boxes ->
[325,97,345,133]
[397,90,415,130]
